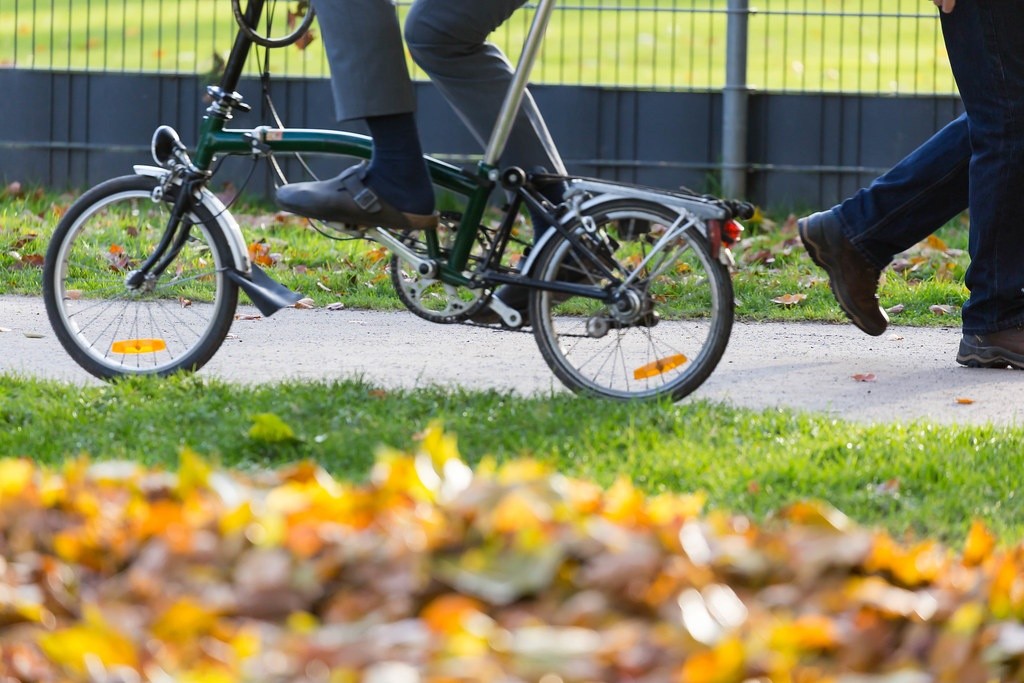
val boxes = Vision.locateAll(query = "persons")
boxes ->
[276,0,613,322]
[798,0,1024,370]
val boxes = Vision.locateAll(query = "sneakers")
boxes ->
[797,208,888,337]
[956,326,1024,370]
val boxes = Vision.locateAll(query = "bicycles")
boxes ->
[42,0,755,407]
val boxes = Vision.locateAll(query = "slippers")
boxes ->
[467,243,614,322]
[275,159,440,230]
